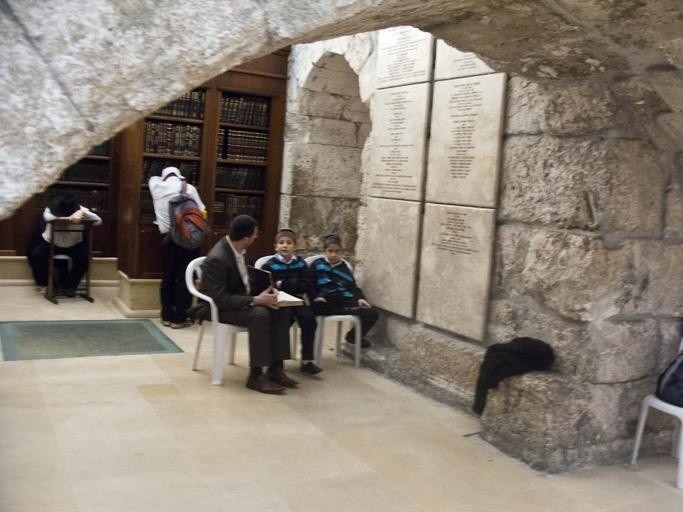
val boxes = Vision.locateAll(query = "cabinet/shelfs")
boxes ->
[114,65,275,282]
[19,127,117,261]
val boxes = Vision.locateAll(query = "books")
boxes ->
[143,123,201,156]
[216,167,264,192]
[219,93,270,127]
[217,196,263,213]
[216,129,268,163]
[149,88,206,119]
[142,159,201,186]
[248,264,303,307]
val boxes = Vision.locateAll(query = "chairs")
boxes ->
[184,258,253,388]
[255,258,319,359]
[304,254,364,370]
[629,337,682,487]
[42,218,97,303]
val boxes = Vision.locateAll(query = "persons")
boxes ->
[29,193,101,296]
[307,233,377,349]
[260,227,322,377]
[149,168,208,327]
[187,215,300,393]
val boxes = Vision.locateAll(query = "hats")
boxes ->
[162,167,184,180]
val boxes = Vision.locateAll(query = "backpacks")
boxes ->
[168,179,212,251]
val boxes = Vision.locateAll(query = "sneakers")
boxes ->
[160,320,169,325]
[299,360,323,375]
[268,366,300,388]
[344,330,372,349]
[170,320,192,329]
[245,372,285,393]
